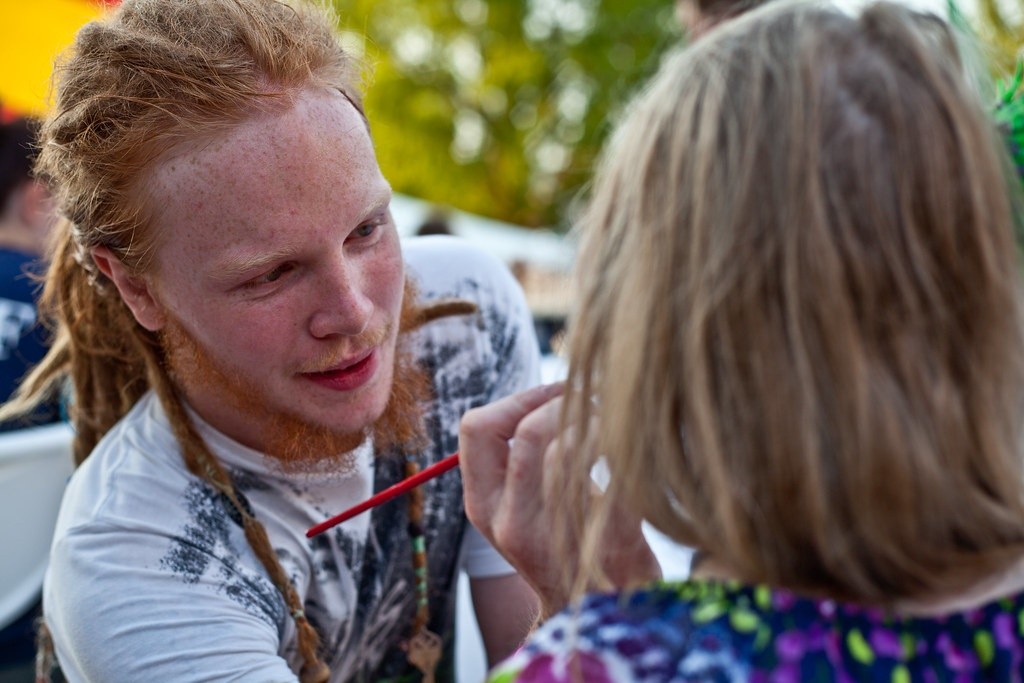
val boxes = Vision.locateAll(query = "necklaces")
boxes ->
[162,408,445,683]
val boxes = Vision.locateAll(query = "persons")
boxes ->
[0,0,660,683]
[0,115,90,432]
[486,0,1021,683]
